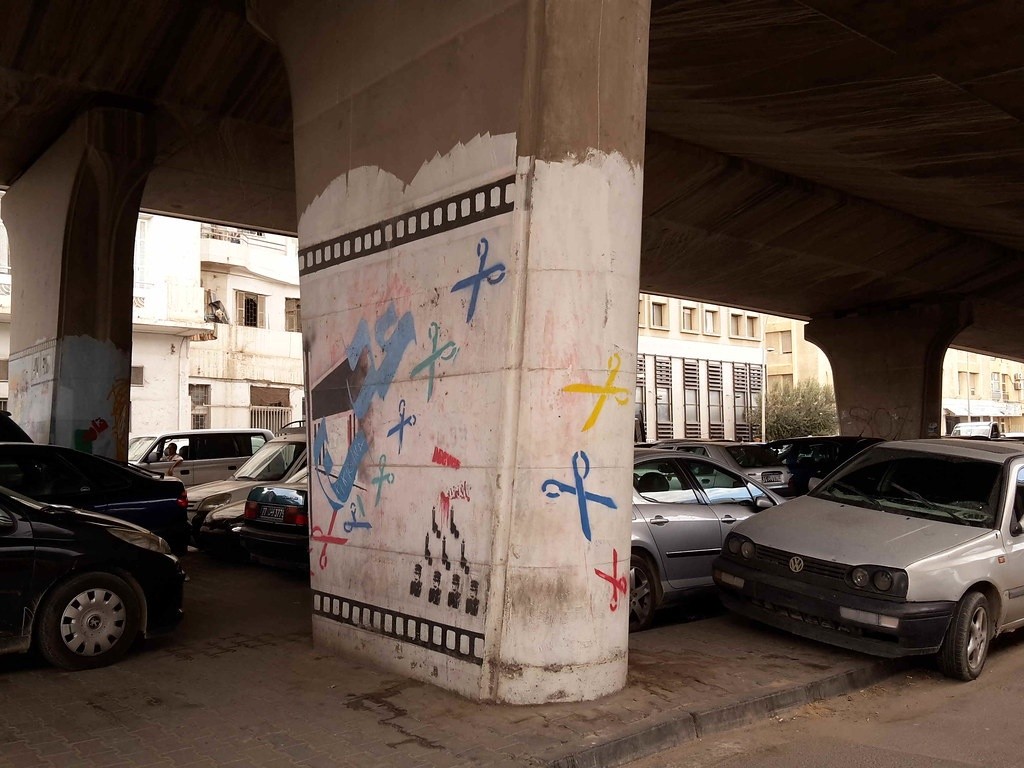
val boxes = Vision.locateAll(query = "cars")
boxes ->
[766,435,888,498]
[0,409,34,441]
[0,439,191,557]
[179,433,309,550]
[233,468,309,560]
[633,438,791,492]
[712,426,1024,682]
[0,483,186,669]
[630,448,788,632]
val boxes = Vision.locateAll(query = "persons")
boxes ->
[160,442,184,476]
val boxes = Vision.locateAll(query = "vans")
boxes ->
[951,420,1002,439]
[127,428,288,492]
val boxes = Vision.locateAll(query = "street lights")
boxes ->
[760,347,775,444]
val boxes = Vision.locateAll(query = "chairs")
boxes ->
[638,472,670,493]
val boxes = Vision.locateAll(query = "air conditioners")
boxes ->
[1014,383,1022,390]
[1014,373,1022,380]
